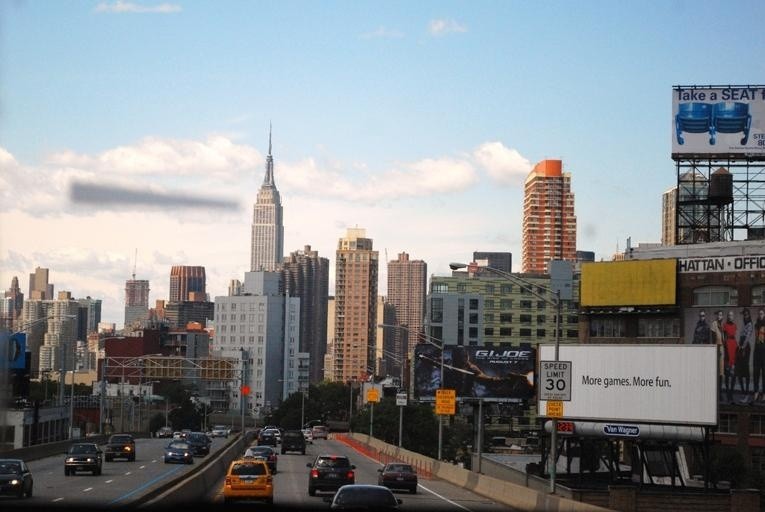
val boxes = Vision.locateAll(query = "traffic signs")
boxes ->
[538,358,572,402]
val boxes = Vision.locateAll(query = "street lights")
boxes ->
[203,394,224,430]
[303,419,322,427]
[377,322,445,461]
[277,378,305,430]
[321,343,405,446]
[448,261,564,494]
[0,314,77,451]
[68,335,124,439]
[163,395,181,427]
[99,357,145,434]
[417,353,482,476]
[137,380,161,432]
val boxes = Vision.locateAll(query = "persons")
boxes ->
[692,307,711,345]
[722,311,740,398]
[709,310,726,404]
[734,307,754,404]
[441,345,505,398]
[750,307,765,403]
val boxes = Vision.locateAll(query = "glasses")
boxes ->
[699,315,705,317]
[744,314,747,316]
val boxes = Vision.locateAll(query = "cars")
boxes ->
[164,440,194,464]
[377,463,418,494]
[156,424,242,440]
[257,425,327,456]
[186,432,212,458]
[323,484,403,512]
[1,459,33,498]
[244,446,279,475]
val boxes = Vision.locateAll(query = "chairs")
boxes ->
[713,101,752,145]
[674,102,713,145]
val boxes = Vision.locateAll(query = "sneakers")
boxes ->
[739,395,751,404]
[761,393,765,403]
[720,394,725,401]
[754,392,761,401]
[726,392,737,404]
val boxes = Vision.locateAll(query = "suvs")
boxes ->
[104,433,137,461]
[306,454,358,496]
[224,460,274,505]
[63,443,104,477]
